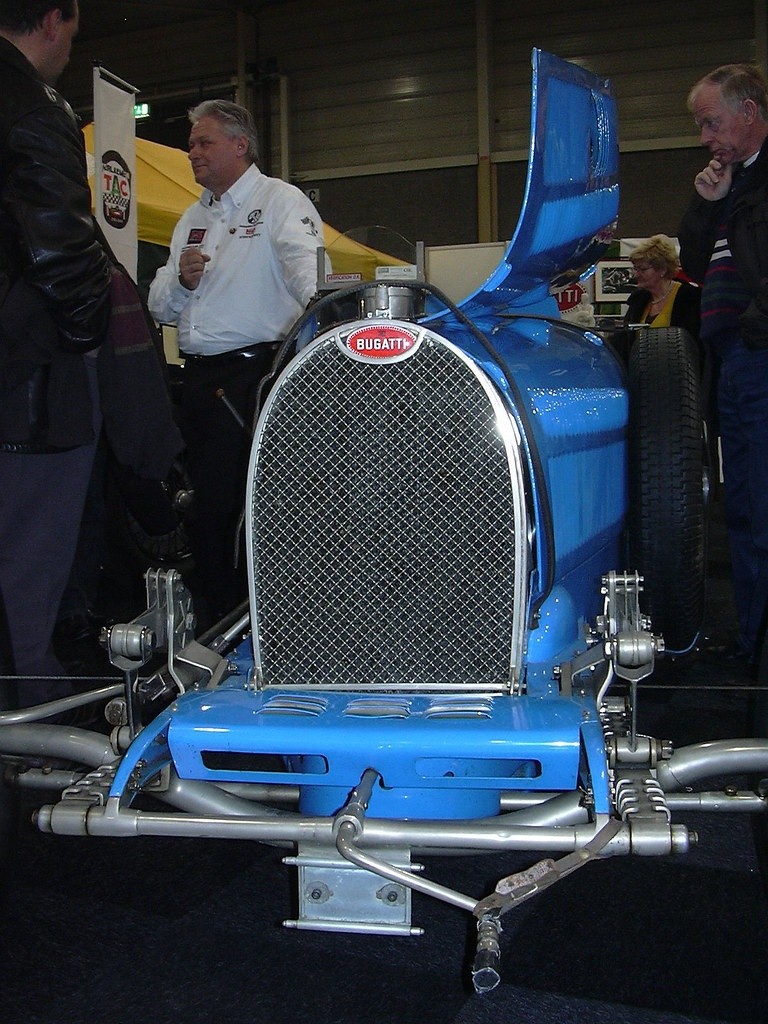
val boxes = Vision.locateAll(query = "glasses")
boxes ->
[632,266,655,273]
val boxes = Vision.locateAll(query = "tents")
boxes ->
[83,117,426,291]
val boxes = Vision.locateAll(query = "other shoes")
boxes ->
[47,701,103,727]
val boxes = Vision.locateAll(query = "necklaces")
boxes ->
[651,284,673,304]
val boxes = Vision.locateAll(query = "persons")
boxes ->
[621,235,702,336]
[678,62,768,670]
[0,0,122,731]
[147,99,332,653]
